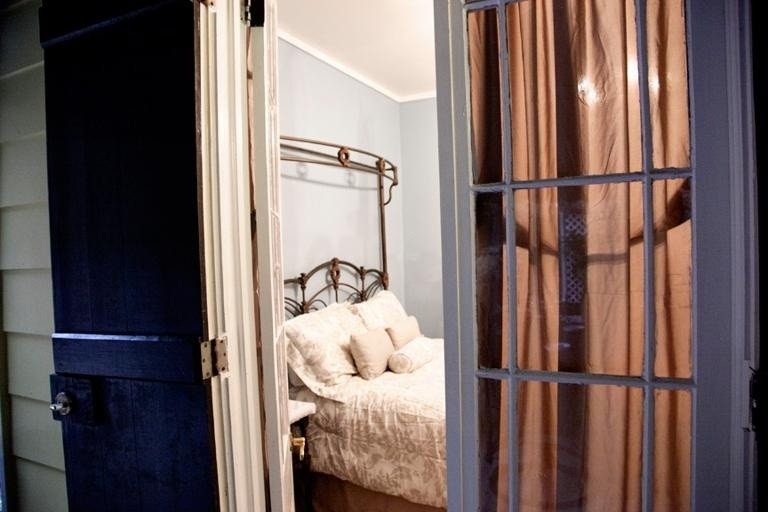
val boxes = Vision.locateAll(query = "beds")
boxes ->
[280,257,448,509]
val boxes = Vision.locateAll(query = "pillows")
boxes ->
[282,287,441,400]
